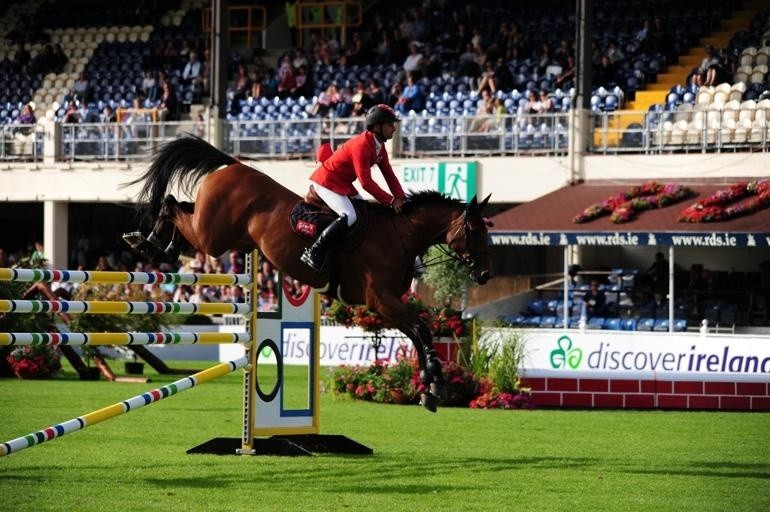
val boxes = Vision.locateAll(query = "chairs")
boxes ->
[496,262,770,332]
[1,2,214,153]
[592,3,770,155]
[221,3,576,155]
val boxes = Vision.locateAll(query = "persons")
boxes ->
[299,103,411,273]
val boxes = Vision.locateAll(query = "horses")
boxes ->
[121,129,495,413]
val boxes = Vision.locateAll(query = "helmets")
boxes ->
[365,103,402,129]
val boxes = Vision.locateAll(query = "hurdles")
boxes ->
[0,249,323,458]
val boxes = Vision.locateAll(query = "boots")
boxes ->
[300,213,349,274]
